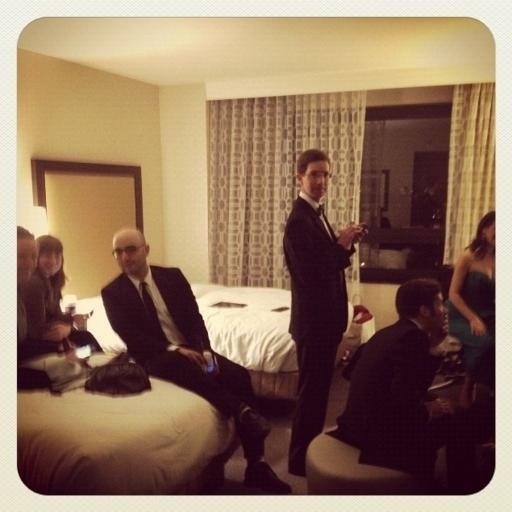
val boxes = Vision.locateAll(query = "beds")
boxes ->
[18,357,249,495]
[54,282,361,407]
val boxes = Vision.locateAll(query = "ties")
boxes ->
[139,280,169,346]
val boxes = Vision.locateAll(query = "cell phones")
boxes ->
[204,355,219,376]
[351,223,368,241]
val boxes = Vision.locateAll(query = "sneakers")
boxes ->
[240,409,271,439]
[244,462,292,494]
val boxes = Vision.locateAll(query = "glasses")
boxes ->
[111,245,146,259]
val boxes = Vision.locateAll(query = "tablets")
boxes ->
[75,343,93,359]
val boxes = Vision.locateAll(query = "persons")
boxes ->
[18,227,71,392]
[445,210,495,408]
[283,149,369,477]
[336,278,468,494]
[30,235,105,353]
[100,227,293,495]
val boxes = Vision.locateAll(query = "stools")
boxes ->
[307,421,413,494]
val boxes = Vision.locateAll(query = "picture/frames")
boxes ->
[360,168,390,212]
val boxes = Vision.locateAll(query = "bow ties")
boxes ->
[317,203,326,216]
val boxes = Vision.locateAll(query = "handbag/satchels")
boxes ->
[84,361,152,396]
